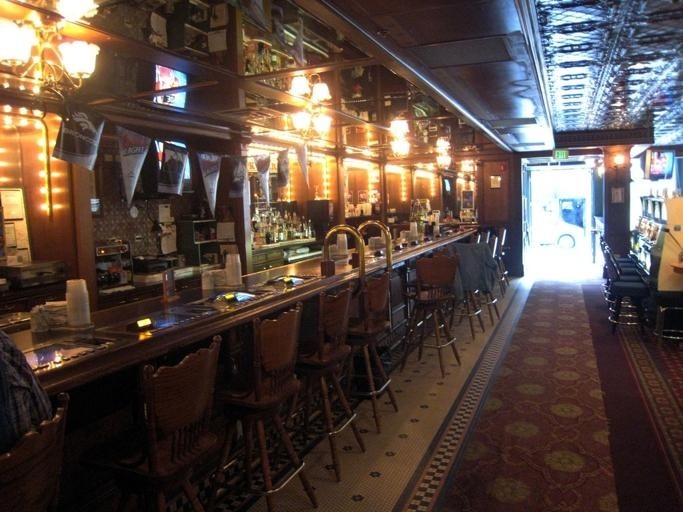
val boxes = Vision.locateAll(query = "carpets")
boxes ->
[393,279,683,512]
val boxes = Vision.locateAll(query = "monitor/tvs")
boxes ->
[135,63,190,113]
[641,147,675,180]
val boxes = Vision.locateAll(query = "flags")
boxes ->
[158,143,189,200]
[229,157,254,198]
[51,101,106,173]
[276,150,290,188]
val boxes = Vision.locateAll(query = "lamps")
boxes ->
[0,19,101,90]
[12,0,506,155]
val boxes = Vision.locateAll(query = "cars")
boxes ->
[534,195,590,250]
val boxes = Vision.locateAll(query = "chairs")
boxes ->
[597,234,649,322]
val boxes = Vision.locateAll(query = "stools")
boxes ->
[609,281,647,335]
[0,225,510,512]
[652,290,683,348]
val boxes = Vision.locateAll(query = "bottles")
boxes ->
[251,209,317,245]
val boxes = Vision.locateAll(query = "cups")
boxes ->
[224,253,242,286]
[337,233,348,256]
[65,278,91,328]
